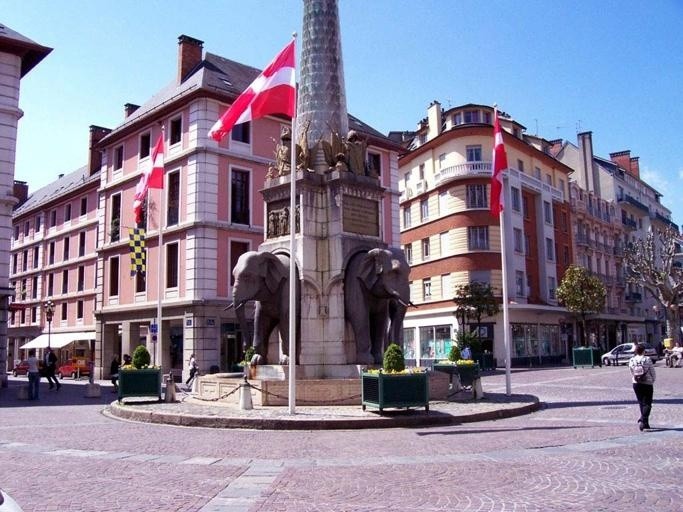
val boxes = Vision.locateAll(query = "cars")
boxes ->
[58,358,93,379]
[13,360,44,377]
[600,342,659,365]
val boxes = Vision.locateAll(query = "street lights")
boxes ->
[43,300,56,347]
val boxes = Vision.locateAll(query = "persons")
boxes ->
[662,342,683,368]
[42,347,61,391]
[109,354,119,390]
[27,349,40,400]
[185,353,198,386]
[628,344,654,431]
[460,344,471,359]
[123,354,131,365]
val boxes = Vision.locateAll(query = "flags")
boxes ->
[206,39,297,142]
[126,228,146,282]
[136,133,164,196]
[132,174,146,224]
[488,111,510,217]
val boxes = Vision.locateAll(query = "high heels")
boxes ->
[638,418,650,431]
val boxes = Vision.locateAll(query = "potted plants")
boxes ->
[118,345,163,402]
[238,347,259,379]
[431,346,481,389]
[362,343,430,414]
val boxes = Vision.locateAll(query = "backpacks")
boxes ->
[633,364,651,381]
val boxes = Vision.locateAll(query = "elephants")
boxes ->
[345,248,419,364]
[223,251,301,366]
